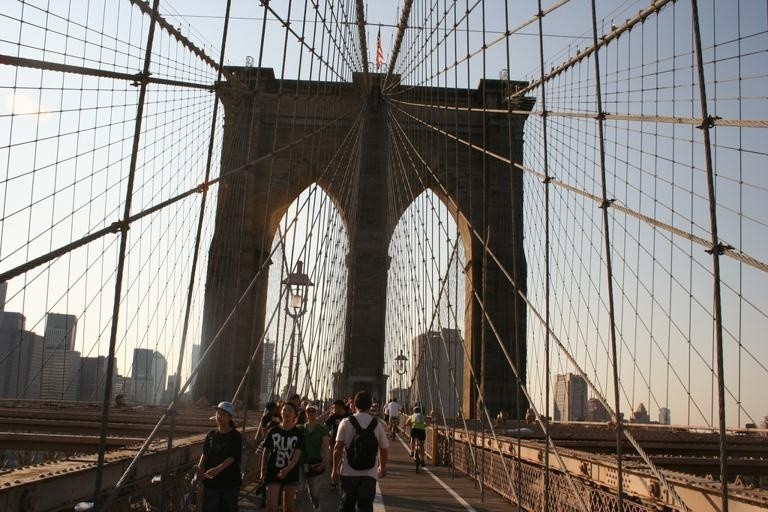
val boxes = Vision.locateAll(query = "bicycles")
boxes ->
[389,416,398,440]
[413,435,422,475]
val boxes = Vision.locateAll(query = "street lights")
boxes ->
[281,261,316,407]
[394,350,409,414]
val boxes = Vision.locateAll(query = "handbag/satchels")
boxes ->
[304,461,325,476]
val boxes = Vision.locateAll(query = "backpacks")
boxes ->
[414,413,422,423]
[347,415,378,470]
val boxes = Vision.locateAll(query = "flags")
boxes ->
[376,31,384,70]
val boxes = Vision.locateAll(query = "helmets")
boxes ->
[413,407,422,413]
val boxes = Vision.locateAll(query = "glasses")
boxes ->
[307,409,315,413]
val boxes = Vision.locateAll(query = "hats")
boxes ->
[306,404,319,411]
[214,401,235,416]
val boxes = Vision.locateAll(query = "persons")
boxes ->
[189,401,243,511]
[253,391,403,512]
[404,406,432,467]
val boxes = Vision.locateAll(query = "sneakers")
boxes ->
[409,451,414,456]
[313,504,320,511]
[420,459,424,465]
